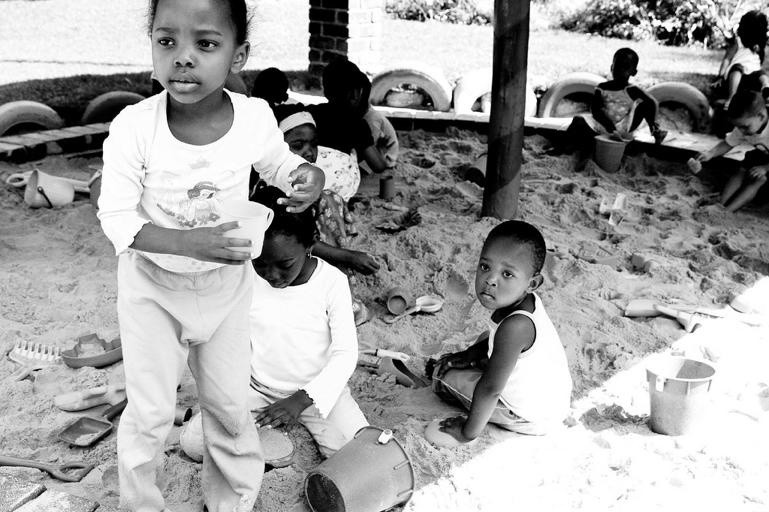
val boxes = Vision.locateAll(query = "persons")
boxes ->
[688,11,769,213]
[575,48,667,172]
[425,220,573,443]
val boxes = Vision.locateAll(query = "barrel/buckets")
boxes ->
[303,426,415,512]
[592,135,627,171]
[23,167,76,209]
[645,356,716,435]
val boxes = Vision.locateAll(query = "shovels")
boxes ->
[58,399,128,447]
